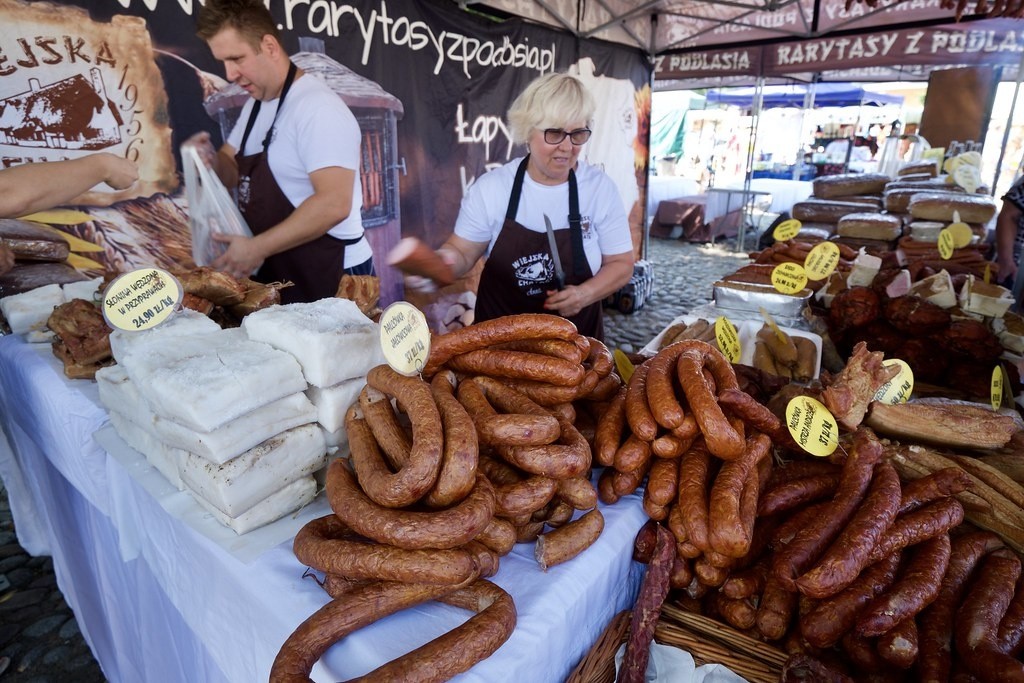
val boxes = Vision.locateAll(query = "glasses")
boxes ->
[544,125,592,146]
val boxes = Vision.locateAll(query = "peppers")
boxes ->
[660,317,817,387]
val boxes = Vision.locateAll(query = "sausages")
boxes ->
[386,237,456,284]
[721,236,995,296]
[269,311,621,683]
[589,336,1024,683]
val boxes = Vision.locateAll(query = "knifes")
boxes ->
[544,214,567,293]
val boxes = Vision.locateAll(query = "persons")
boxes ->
[404,73,634,342]
[0,152,141,218]
[180,0,375,303]
[895,122,1024,291]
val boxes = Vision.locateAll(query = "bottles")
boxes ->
[601,260,654,314]
[940,140,982,175]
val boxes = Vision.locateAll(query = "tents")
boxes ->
[651,81,907,182]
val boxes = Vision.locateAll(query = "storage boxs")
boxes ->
[911,269,955,309]
[645,313,823,386]
[959,278,1015,319]
[712,279,812,317]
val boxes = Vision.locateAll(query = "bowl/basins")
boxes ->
[910,222,944,242]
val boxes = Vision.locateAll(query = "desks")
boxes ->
[709,187,772,249]
[0,332,650,683]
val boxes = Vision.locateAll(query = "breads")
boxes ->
[789,160,995,243]
